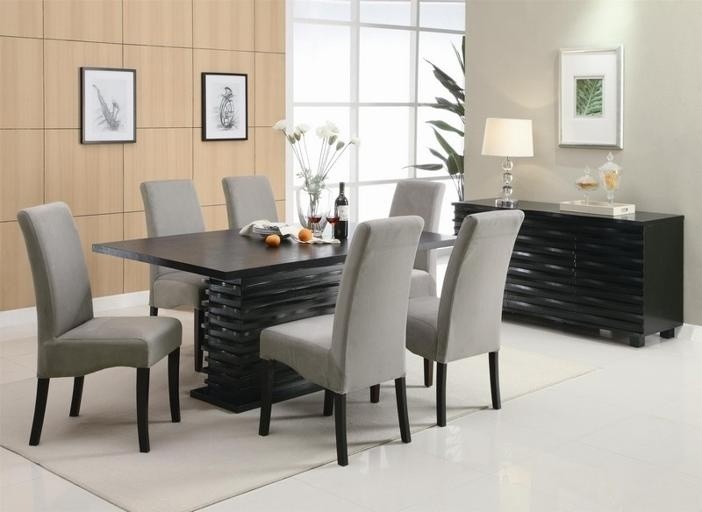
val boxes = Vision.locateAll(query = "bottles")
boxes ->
[598,152,619,202]
[576,167,598,202]
[335,181,349,239]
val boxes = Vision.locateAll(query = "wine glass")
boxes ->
[306,199,322,236]
[324,201,338,242]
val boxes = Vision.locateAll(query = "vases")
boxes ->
[295,177,333,239]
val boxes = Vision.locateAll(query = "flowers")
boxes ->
[272,119,360,232]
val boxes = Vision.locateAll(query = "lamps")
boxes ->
[481,118,534,209]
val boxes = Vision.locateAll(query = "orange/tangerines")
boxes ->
[298,229,313,242]
[265,234,281,246]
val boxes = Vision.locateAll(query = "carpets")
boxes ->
[1,290,602,510]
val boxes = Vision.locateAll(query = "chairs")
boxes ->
[222,175,278,229]
[407,210,526,427]
[389,179,446,299]
[258,215,424,467]
[139,179,210,372]
[16,201,183,453]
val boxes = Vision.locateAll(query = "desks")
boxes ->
[92,222,458,414]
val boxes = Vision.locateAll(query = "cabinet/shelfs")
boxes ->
[452,198,685,347]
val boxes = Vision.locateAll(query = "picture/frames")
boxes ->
[80,66,136,145]
[201,72,248,142]
[558,46,625,150]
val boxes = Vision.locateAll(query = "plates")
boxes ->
[251,223,291,241]
